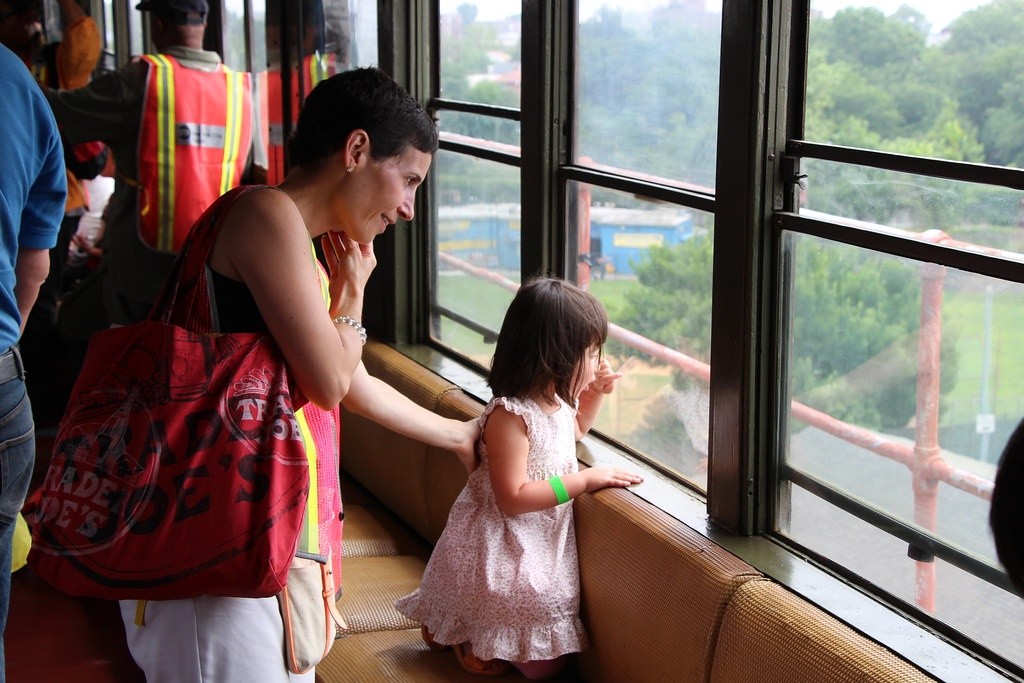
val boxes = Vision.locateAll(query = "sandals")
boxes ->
[420,622,448,652]
[452,642,509,678]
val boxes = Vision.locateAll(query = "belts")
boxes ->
[1,350,22,387]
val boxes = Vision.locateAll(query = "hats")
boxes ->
[136,0,224,25]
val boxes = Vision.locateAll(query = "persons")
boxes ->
[117,64,482,682]
[392,270,646,680]
[1,2,255,330]
[0,41,69,683]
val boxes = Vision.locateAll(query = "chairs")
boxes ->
[317,341,936,683]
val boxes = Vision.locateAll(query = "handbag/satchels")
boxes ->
[26,183,312,602]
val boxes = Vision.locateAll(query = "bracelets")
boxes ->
[546,473,573,506]
[331,316,368,345]
